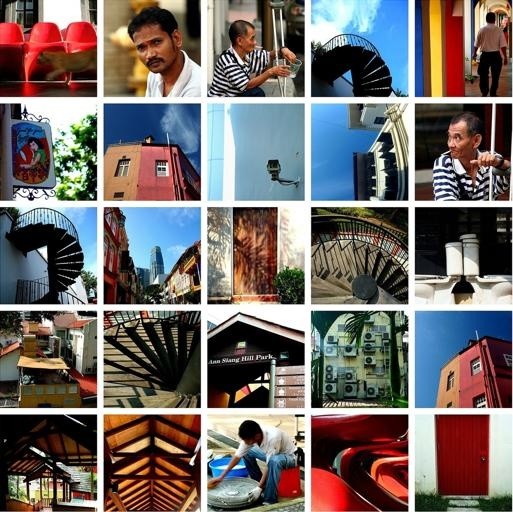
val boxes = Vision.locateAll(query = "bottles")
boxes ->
[444,238,480,275]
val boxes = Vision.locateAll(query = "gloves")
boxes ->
[248,486,262,504]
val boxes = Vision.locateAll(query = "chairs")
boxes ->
[0,20,97,86]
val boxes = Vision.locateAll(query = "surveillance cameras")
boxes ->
[267,159,281,181]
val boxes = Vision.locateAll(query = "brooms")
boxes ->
[450,242,475,295]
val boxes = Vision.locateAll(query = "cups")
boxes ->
[286,58,302,79]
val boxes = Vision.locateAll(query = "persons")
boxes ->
[209,415,299,505]
[471,10,507,96]
[207,19,298,97]
[428,110,510,201]
[125,8,201,100]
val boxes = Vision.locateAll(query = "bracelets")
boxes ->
[494,153,504,171]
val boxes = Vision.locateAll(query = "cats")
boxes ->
[36,49,97,85]
